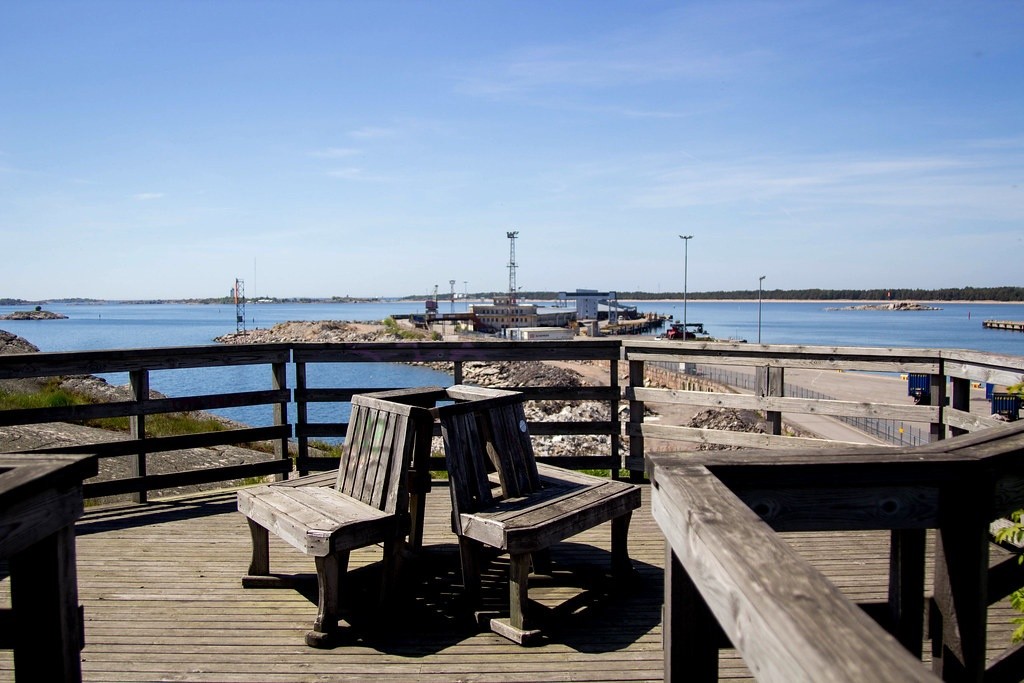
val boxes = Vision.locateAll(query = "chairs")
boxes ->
[237,385,642,647]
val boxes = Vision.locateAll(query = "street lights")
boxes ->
[462,281,470,311]
[678,235,694,342]
[758,275,766,343]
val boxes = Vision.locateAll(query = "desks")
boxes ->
[0,452,99,683]
